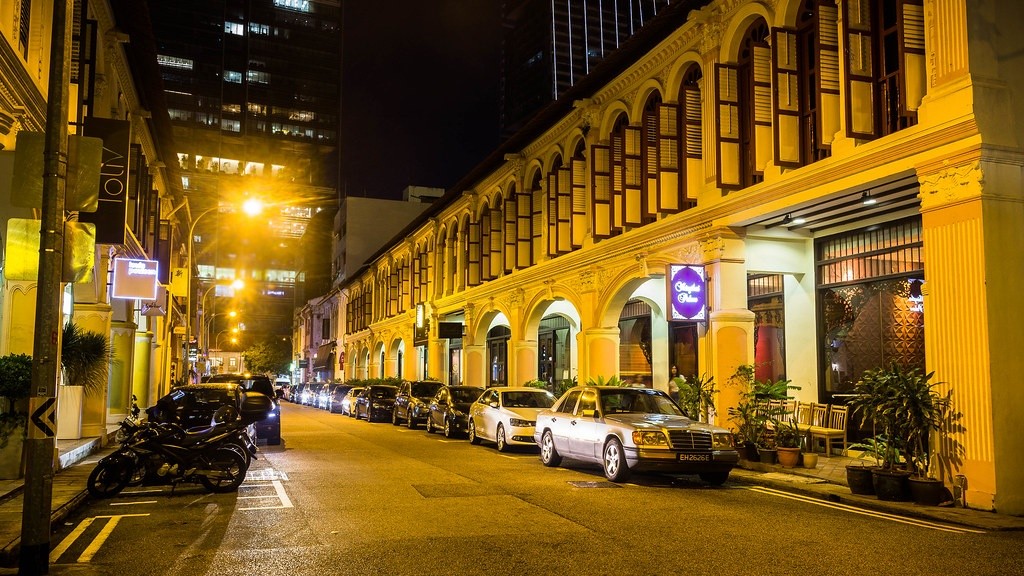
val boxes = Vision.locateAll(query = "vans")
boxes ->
[273,378,290,396]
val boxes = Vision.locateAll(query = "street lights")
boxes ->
[183,198,262,386]
[216,328,238,360]
[200,279,245,356]
[217,338,237,359]
[207,311,236,367]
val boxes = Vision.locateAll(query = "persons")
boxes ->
[632,374,645,387]
[670,364,686,400]
[277,388,285,398]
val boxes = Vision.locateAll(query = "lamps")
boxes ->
[863,190,877,205]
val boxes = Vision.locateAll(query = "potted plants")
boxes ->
[843,358,955,503]
[57,320,120,440]
[723,362,805,467]
[0,352,33,480]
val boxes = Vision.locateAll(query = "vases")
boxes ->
[801,452,818,468]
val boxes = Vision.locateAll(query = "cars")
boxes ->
[205,372,284,446]
[282,381,357,413]
[355,385,397,422]
[170,383,247,431]
[391,379,447,429]
[427,385,500,438]
[533,385,741,485]
[341,387,366,417]
[467,386,558,452]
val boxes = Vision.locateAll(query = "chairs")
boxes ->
[770,400,797,429]
[793,403,829,452]
[759,399,783,426]
[810,404,849,458]
[788,401,814,452]
[758,399,770,417]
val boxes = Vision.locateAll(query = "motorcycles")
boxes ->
[86,395,267,499]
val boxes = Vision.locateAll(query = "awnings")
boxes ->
[313,343,333,372]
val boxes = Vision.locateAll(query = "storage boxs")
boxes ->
[240,391,272,420]
